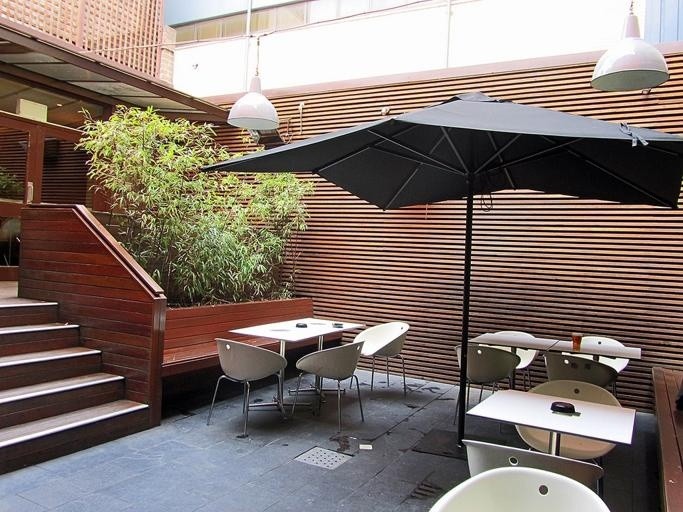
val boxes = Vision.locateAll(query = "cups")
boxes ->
[570,332,584,350]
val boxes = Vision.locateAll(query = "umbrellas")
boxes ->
[201,89,682,449]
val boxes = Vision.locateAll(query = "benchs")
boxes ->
[651,365,682,512]
[163,298,342,374]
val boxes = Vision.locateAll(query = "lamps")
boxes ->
[223,32,281,132]
[584,1,675,96]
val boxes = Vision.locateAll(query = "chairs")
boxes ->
[206,316,410,433]
[429,328,641,510]
[0,213,20,266]
